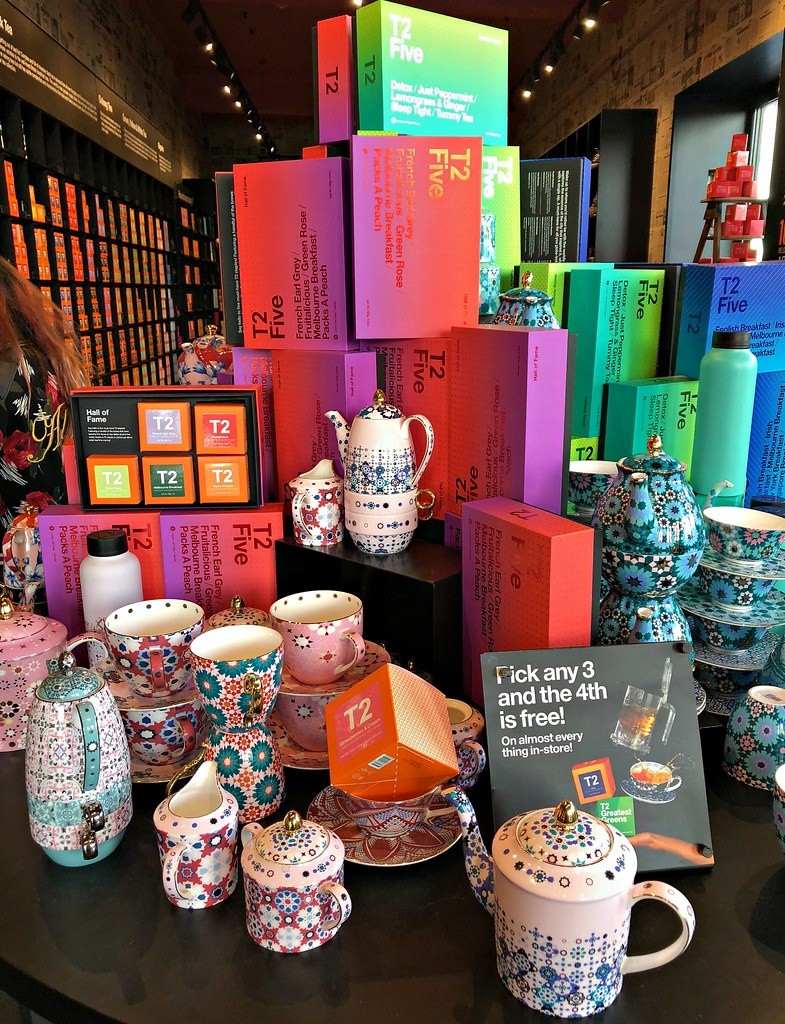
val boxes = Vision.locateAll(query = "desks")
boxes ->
[0,730,785,1024]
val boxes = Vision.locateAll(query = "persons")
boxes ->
[0,256,92,613]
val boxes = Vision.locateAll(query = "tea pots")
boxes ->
[322,390,434,556]
[176,324,234,385]
[586,433,734,672]
[3,505,45,590]
[25,652,133,867]
[1,598,111,752]
[490,271,561,330]
[440,786,696,1019]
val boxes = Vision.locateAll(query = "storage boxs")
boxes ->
[137,401,192,452]
[71,384,266,512]
[270,348,376,517]
[311,14,353,144]
[197,454,251,504]
[352,0,508,146]
[325,662,459,801]
[302,143,349,158]
[445,134,785,515]
[142,455,196,505]
[462,496,595,706]
[350,136,482,339]
[37,504,165,641]
[359,339,451,521]
[233,158,360,351]
[86,454,143,506]
[160,502,283,620]
[193,404,247,454]
[215,172,245,347]
[233,346,279,502]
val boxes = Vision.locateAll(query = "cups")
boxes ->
[721,686,785,791]
[773,762,785,855]
[444,697,485,788]
[189,625,285,733]
[240,811,352,953]
[276,694,347,751]
[119,700,207,766]
[610,685,676,753]
[269,590,366,686]
[630,762,682,796]
[203,725,286,824]
[105,599,205,698]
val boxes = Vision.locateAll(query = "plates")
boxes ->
[306,785,463,867]
[699,538,785,581]
[128,748,204,783]
[265,709,331,770]
[620,780,677,804]
[277,641,390,697]
[675,565,785,627]
[88,657,200,711]
[681,608,781,671]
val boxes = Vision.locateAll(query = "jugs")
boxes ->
[289,459,344,547]
[152,761,239,910]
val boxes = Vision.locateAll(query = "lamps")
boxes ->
[224,78,239,94]
[260,140,274,153]
[526,80,535,92]
[530,64,541,82]
[584,1,600,29]
[255,124,266,141]
[590,0,610,6]
[247,108,257,124]
[217,65,235,80]
[547,53,559,68]
[211,48,224,66]
[195,27,213,52]
[234,90,248,109]
[572,25,584,40]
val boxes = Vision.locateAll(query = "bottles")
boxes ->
[691,331,758,497]
[79,529,143,669]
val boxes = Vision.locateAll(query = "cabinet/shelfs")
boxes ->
[692,197,769,263]
[0,87,224,386]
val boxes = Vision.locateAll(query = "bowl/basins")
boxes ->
[567,461,618,513]
[702,506,785,569]
[700,566,778,613]
[691,613,767,656]
[339,787,441,837]
